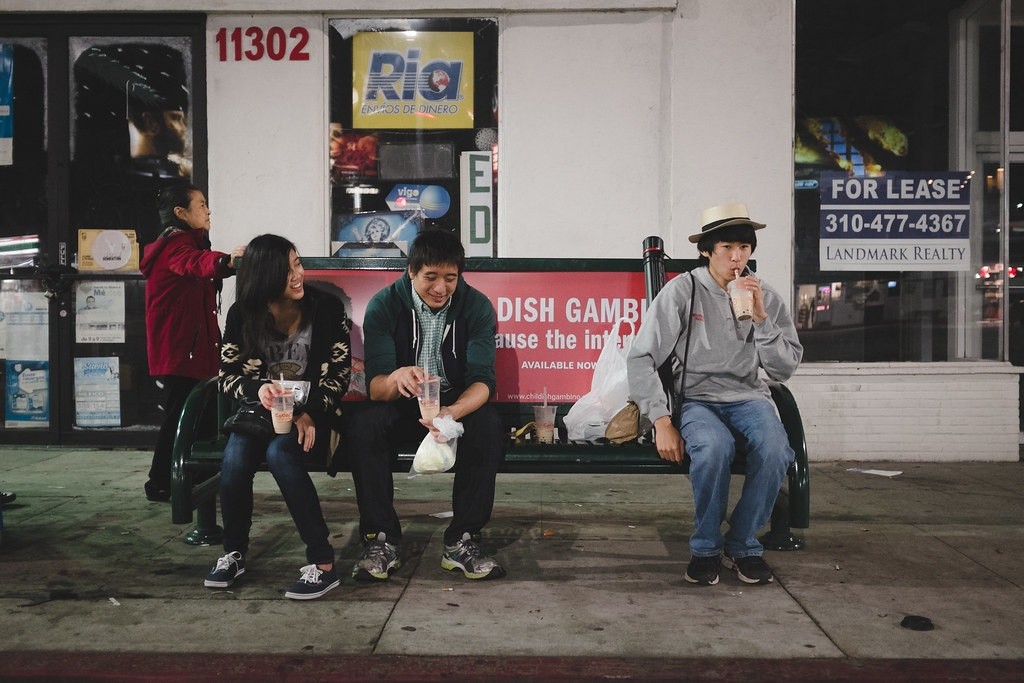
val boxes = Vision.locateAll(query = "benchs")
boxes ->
[170,255,811,551]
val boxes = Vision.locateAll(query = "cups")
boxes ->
[532,405,558,446]
[727,278,754,323]
[413,376,440,422]
[270,391,295,434]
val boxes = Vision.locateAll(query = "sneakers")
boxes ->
[352,532,409,583]
[684,555,719,585]
[441,528,506,580]
[284,562,342,600]
[721,548,775,584]
[204,551,245,587]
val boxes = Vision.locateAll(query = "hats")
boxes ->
[687,203,766,243]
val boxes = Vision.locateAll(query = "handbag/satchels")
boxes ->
[563,317,635,443]
[223,404,274,441]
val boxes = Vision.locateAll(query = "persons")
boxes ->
[140,183,247,502]
[354,228,505,582]
[1,481,18,505]
[127,72,192,184]
[626,203,804,587]
[203,234,352,600]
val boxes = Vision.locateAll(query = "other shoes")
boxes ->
[144,474,171,500]
